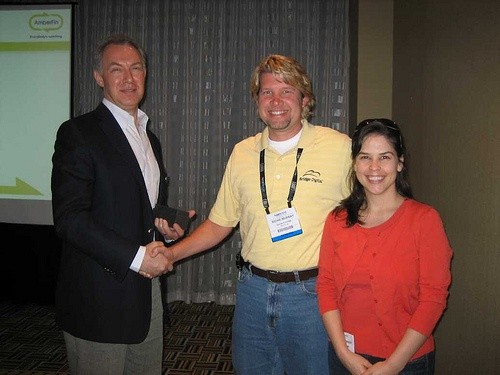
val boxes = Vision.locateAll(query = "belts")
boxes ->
[236,253,319,283]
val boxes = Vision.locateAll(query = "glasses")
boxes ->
[355,119,396,128]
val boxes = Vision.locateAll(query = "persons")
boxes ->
[50,35,197,375]
[315,119,453,375]
[142,55,351,375]
